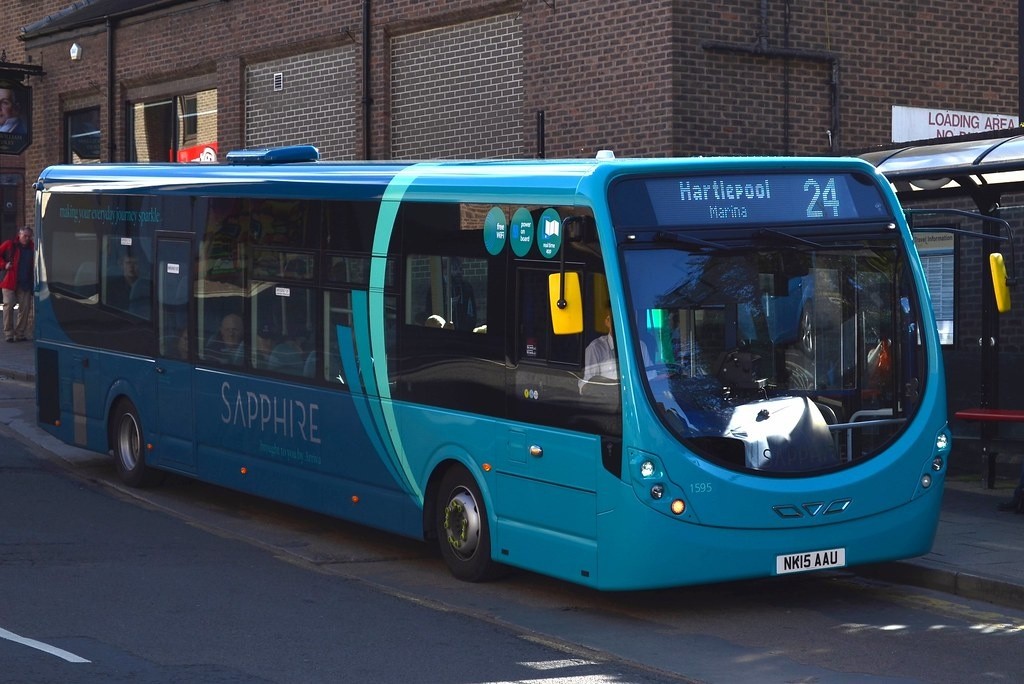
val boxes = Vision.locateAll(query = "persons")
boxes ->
[0,226,35,342]
[110,252,144,308]
[578,315,658,396]
[426,257,477,331]
[169,324,188,360]
[205,314,245,362]
[0,81,26,134]
[256,319,277,355]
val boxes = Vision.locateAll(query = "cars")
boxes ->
[729,256,826,362]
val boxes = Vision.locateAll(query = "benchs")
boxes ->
[955,408,1024,421]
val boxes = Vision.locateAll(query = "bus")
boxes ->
[29,145,1023,588]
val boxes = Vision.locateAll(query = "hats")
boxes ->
[257,319,276,340]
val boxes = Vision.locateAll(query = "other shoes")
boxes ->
[5,334,16,343]
[15,336,27,341]
